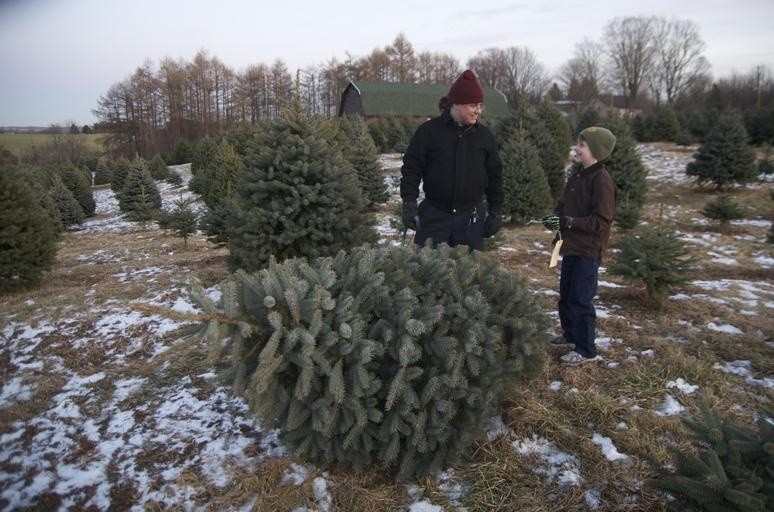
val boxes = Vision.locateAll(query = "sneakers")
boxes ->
[550,335,576,346]
[559,351,597,366]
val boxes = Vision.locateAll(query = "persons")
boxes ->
[543,126,616,366]
[400,68,504,253]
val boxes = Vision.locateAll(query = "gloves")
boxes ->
[403,200,417,231]
[484,209,502,238]
[543,213,574,231]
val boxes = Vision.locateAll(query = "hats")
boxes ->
[579,127,616,161]
[447,70,484,104]
